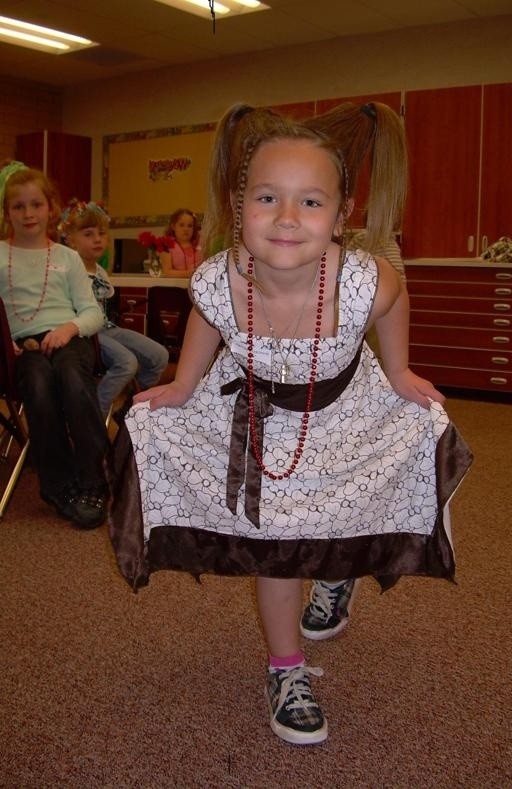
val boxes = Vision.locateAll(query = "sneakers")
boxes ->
[39,481,82,519]
[263,661,330,744]
[75,488,110,531]
[299,574,357,641]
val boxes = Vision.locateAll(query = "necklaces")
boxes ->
[254,265,318,394]
[247,257,325,480]
[8,235,51,322]
[268,324,292,393]
[177,239,196,272]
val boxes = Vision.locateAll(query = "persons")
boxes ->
[55,195,170,428]
[0,154,111,528]
[103,101,472,745]
[158,207,201,275]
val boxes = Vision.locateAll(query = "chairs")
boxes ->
[1,301,139,521]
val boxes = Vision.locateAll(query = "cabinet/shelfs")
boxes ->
[399,262,510,396]
[403,83,511,259]
